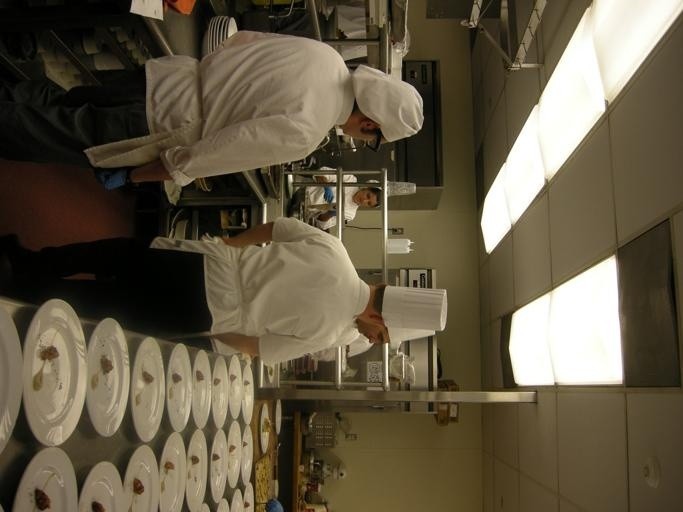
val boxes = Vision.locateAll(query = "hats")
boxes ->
[388,327,435,348]
[351,63,426,143]
[382,284,449,331]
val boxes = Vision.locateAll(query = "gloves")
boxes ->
[323,185,334,204]
[97,166,125,190]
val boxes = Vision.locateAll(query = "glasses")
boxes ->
[365,129,382,155]
